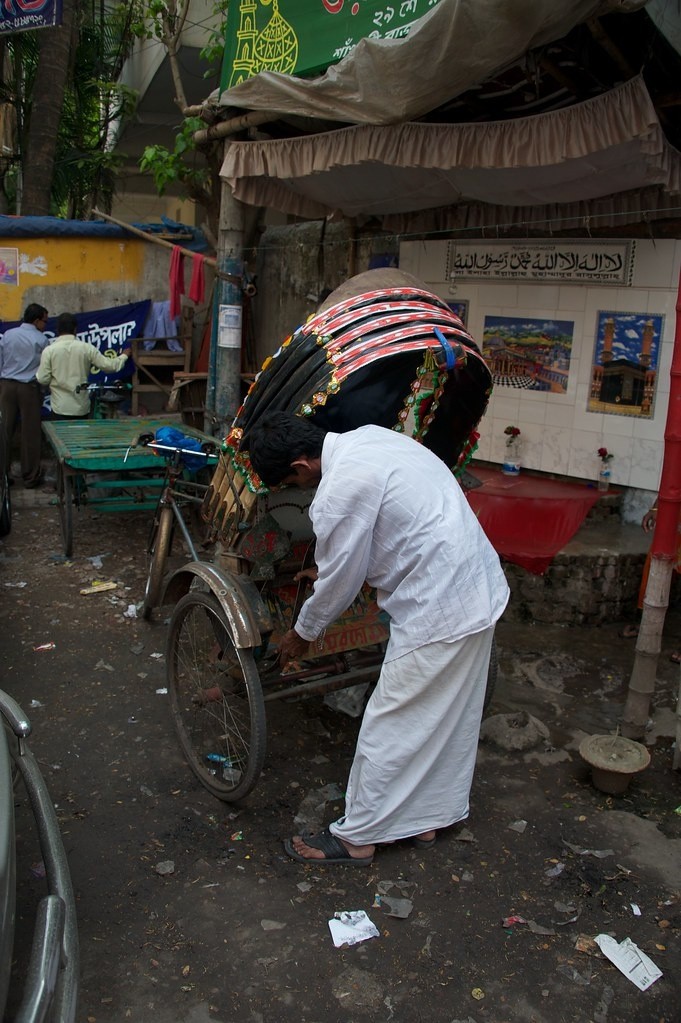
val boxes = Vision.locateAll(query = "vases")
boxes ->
[597,460,610,492]
[503,435,523,476]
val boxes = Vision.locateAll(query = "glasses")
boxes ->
[40,319,48,324]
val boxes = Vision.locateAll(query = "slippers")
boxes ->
[284,828,374,866]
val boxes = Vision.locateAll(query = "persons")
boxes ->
[35,312,135,497]
[247,410,511,867]
[0,302,51,488]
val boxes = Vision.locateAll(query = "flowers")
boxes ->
[503,424,521,438]
[597,447,614,462]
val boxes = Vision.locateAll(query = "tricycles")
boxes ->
[40,373,221,556]
[115,269,494,798]
